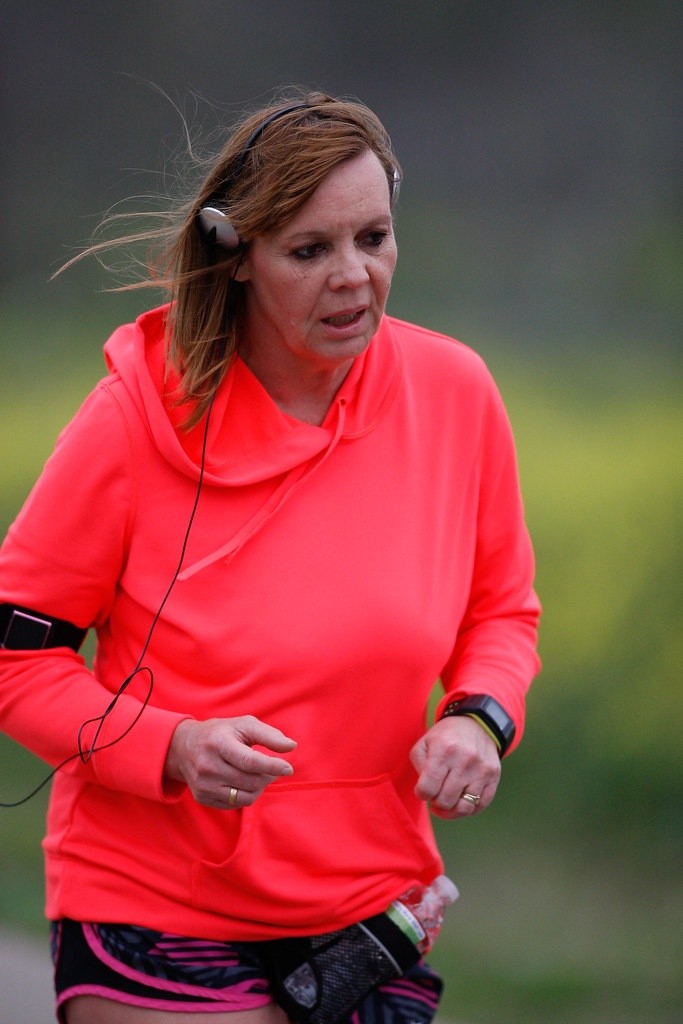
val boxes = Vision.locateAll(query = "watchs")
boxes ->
[438,693,516,760]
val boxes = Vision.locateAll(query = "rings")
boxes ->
[460,792,481,806]
[228,788,238,805]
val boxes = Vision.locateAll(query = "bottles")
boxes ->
[281,874,460,1022]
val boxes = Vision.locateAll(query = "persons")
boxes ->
[0,94,542,1023]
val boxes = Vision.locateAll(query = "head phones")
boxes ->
[197,101,402,251]
